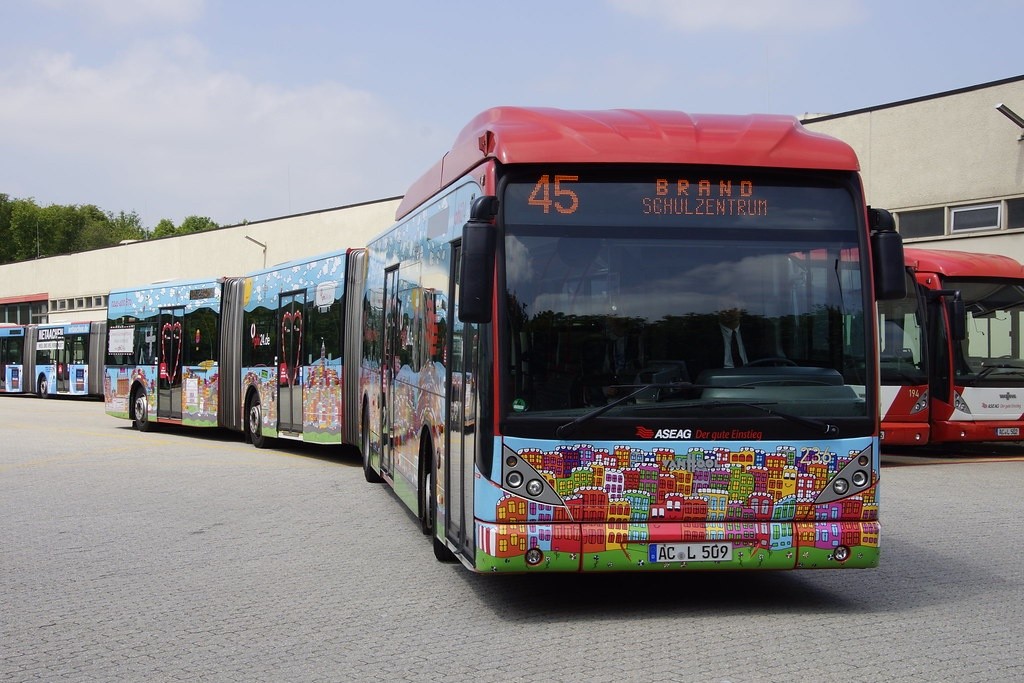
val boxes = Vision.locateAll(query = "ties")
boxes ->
[730,330,743,368]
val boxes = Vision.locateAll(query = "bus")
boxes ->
[103,105,907,579]
[0,321,109,400]
[784,248,1024,451]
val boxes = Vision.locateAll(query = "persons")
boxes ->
[569,310,653,410]
[697,293,786,379]
